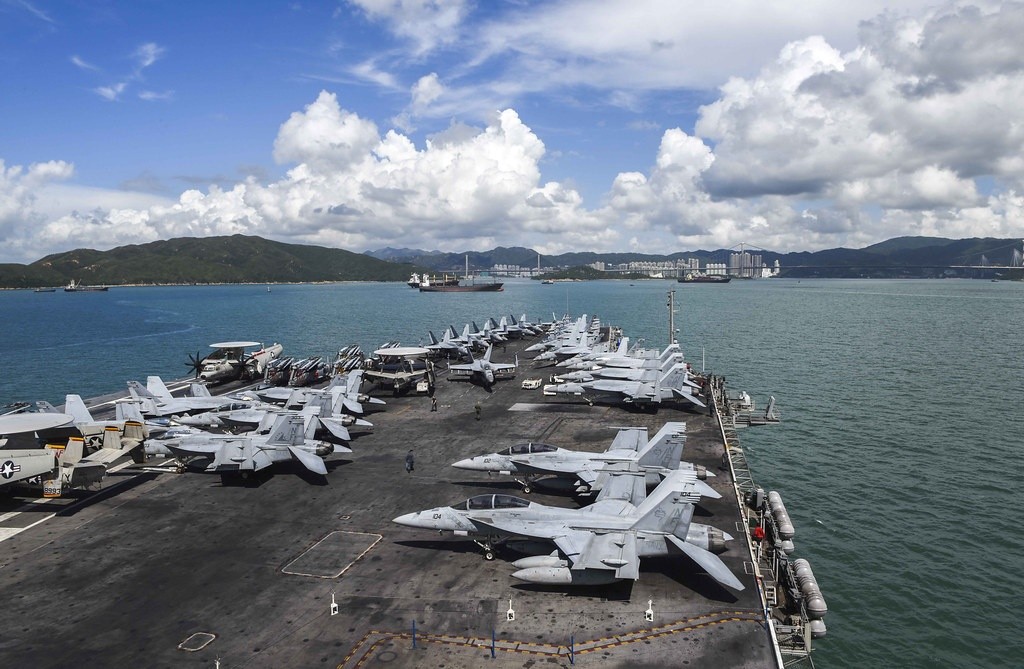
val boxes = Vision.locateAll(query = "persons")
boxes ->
[405,449,414,472]
[474,400,481,419]
[504,344,507,352]
[551,375,555,384]
[752,523,764,546]
[431,394,437,412]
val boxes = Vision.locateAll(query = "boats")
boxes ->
[417,254,505,292]
[35,287,56,292]
[541,281,554,285]
[677,273,732,283]
[407,253,459,288]
[64,278,108,292]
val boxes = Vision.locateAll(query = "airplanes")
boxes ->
[391,466,746,592]
[0,310,708,475]
[451,420,723,499]
